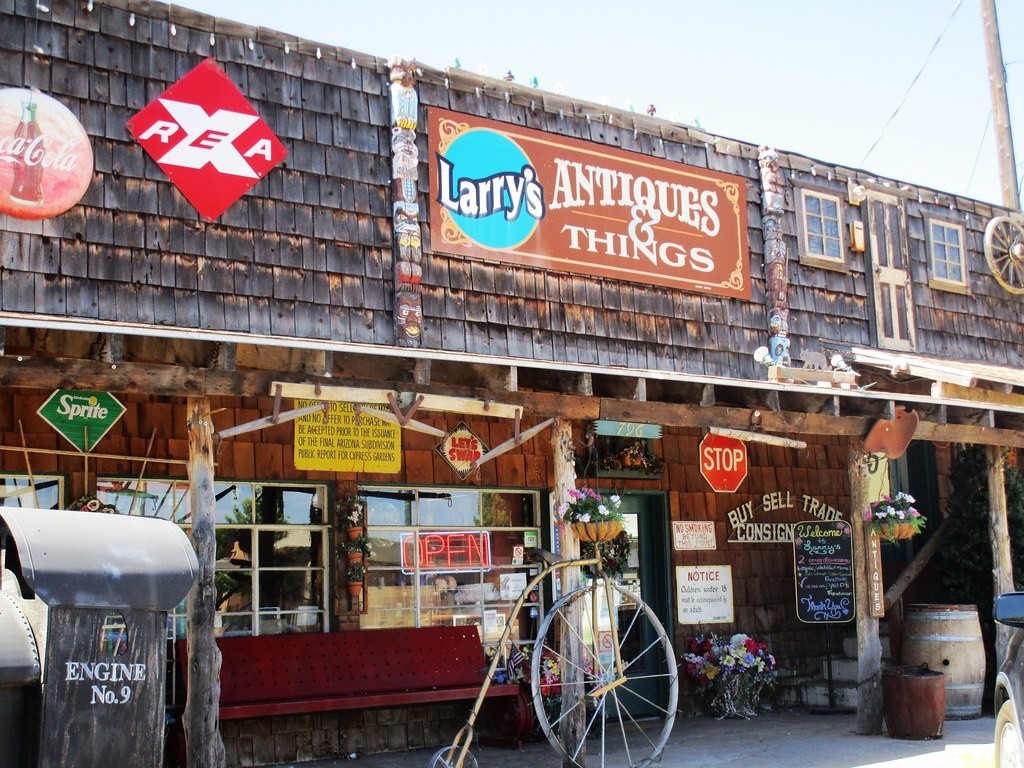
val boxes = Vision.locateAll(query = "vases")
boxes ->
[873,523,917,540]
[570,519,623,543]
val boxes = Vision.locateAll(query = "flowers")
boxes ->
[681,630,776,684]
[562,485,621,522]
[865,491,928,545]
[528,653,601,698]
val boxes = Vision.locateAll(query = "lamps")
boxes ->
[750,345,857,385]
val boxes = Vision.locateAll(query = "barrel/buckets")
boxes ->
[901,604,987,720]
[880,661,945,741]
